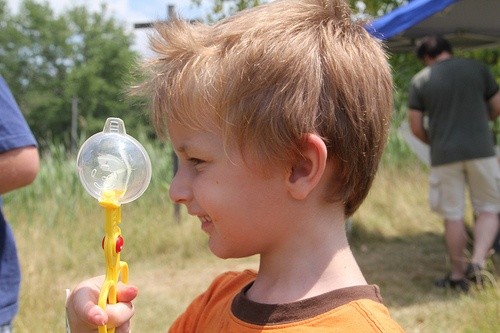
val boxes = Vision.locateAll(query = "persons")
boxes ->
[66,0,407,333]
[407,34,500,292]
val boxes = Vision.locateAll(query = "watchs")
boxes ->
[0,74,39,333]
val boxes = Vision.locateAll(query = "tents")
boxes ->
[363,0,500,54]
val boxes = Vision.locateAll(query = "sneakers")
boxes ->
[465,263,485,285]
[435,274,469,295]
